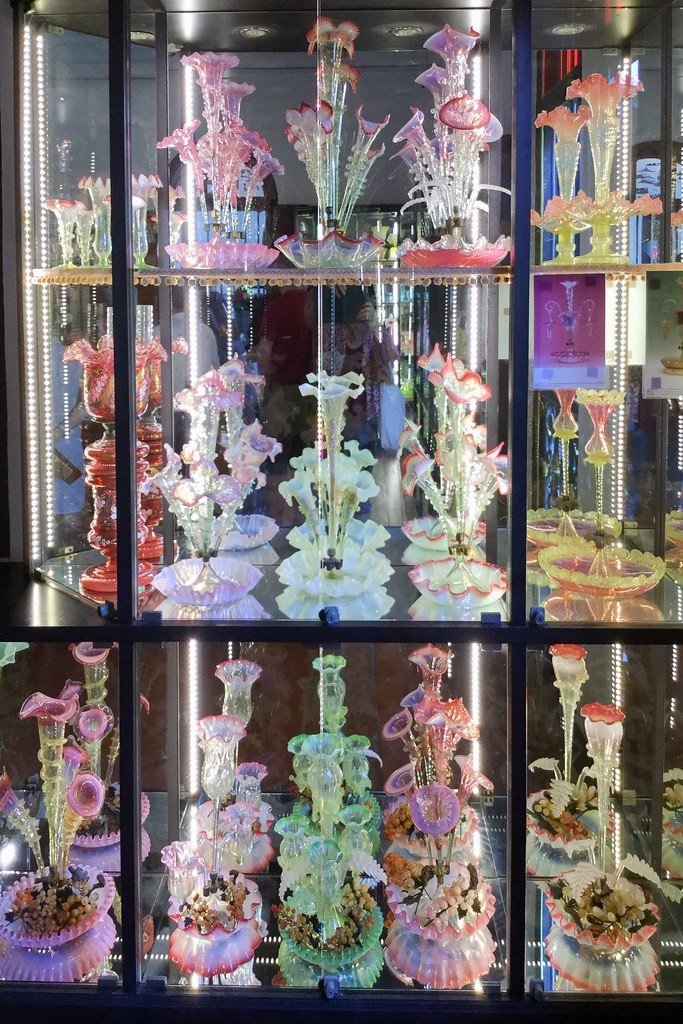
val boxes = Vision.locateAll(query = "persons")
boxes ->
[152,284,419,527]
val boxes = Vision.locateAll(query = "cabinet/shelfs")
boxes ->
[0,0,683,1024]
